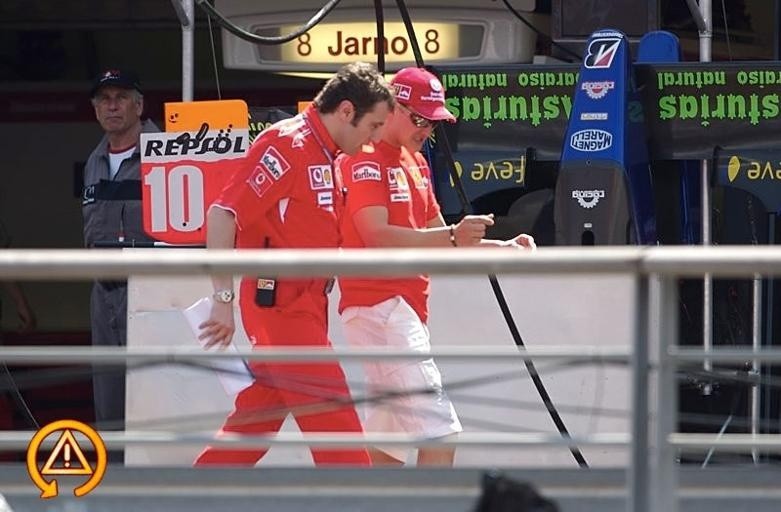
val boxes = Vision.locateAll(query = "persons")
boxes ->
[4,281,38,336]
[336,64,539,472]
[191,63,398,468]
[81,68,165,465]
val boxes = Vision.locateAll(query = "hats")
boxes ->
[389,67,456,125]
[91,64,144,96]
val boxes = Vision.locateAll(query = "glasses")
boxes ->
[395,101,442,129]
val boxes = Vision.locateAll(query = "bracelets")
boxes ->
[449,223,461,248]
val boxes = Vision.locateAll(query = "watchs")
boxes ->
[213,289,237,304]
